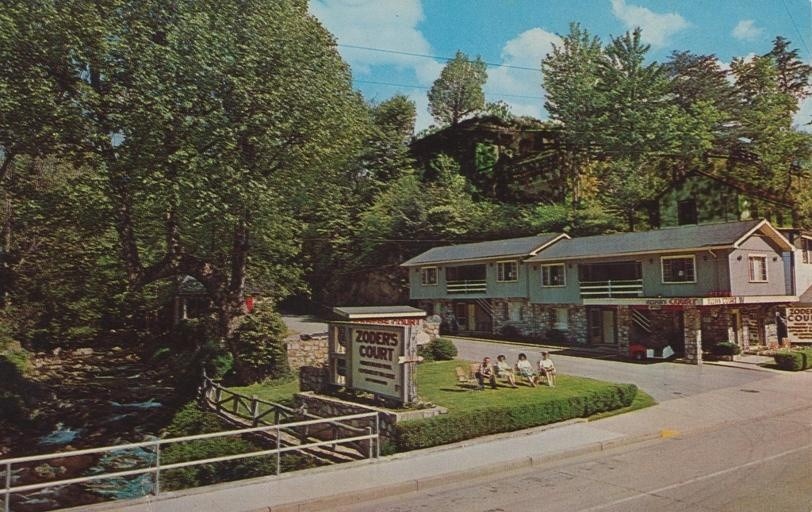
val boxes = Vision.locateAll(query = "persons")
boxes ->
[540,351,556,387]
[478,357,498,390]
[497,355,519,388]
[517,352,540,387]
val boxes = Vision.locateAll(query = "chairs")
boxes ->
[454,362,558,392]
